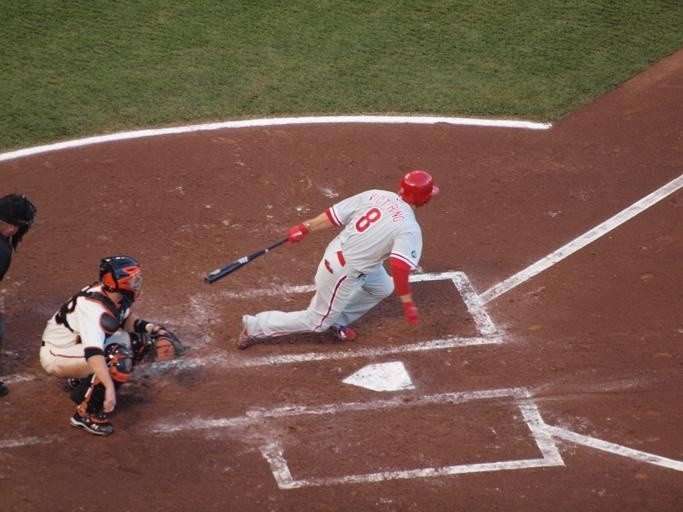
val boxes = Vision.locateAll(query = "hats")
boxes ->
[0,193,36,232]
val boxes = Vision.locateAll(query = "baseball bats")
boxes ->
[205,239,287,284]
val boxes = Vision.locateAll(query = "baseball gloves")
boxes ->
[150,324,185,363]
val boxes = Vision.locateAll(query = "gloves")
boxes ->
[402,300,420,327]
[287,223,309,245]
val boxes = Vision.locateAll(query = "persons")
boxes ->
[237,170,438,350]
[39,255,183,436]
[0,192,36,397]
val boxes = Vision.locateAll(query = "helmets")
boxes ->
[98,256,144,304]
[399,169,440,205]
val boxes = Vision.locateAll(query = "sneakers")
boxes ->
[237,314,250,351]
[69,406,114,437]
[330,323,357,343]
